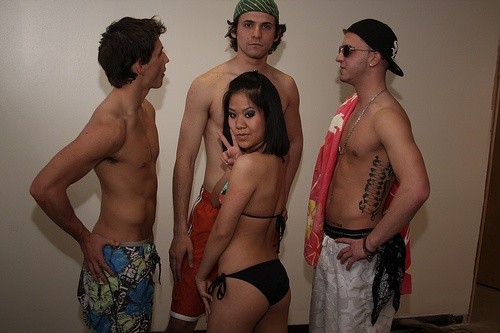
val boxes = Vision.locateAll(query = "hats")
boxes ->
[346,18,404,77]
[233,0,279,21]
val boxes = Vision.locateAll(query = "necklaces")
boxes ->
[339,88,388,158]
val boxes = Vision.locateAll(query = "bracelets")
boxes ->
[364,234,377,261]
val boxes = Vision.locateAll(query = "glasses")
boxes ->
[339,45,376,57]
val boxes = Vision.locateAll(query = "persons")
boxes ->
[165,0,302,333]
[30,14,172,333]
[196,71,290,333]
[304,18,431,333]
